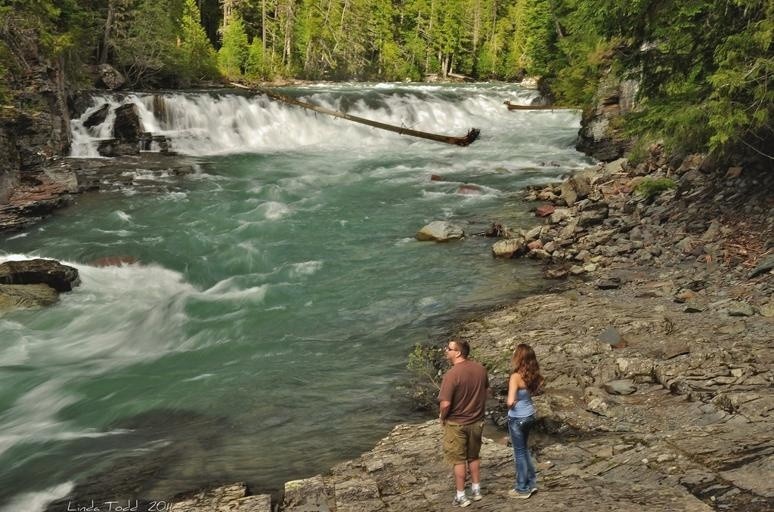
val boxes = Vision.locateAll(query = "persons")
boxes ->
[507,344,545,498]
[438,339,489,509]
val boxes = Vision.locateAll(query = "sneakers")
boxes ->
[452,494,471,508]
[467,488,483,502]
[508,487,539,499]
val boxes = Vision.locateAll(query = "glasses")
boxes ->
[447,348,456,351]
